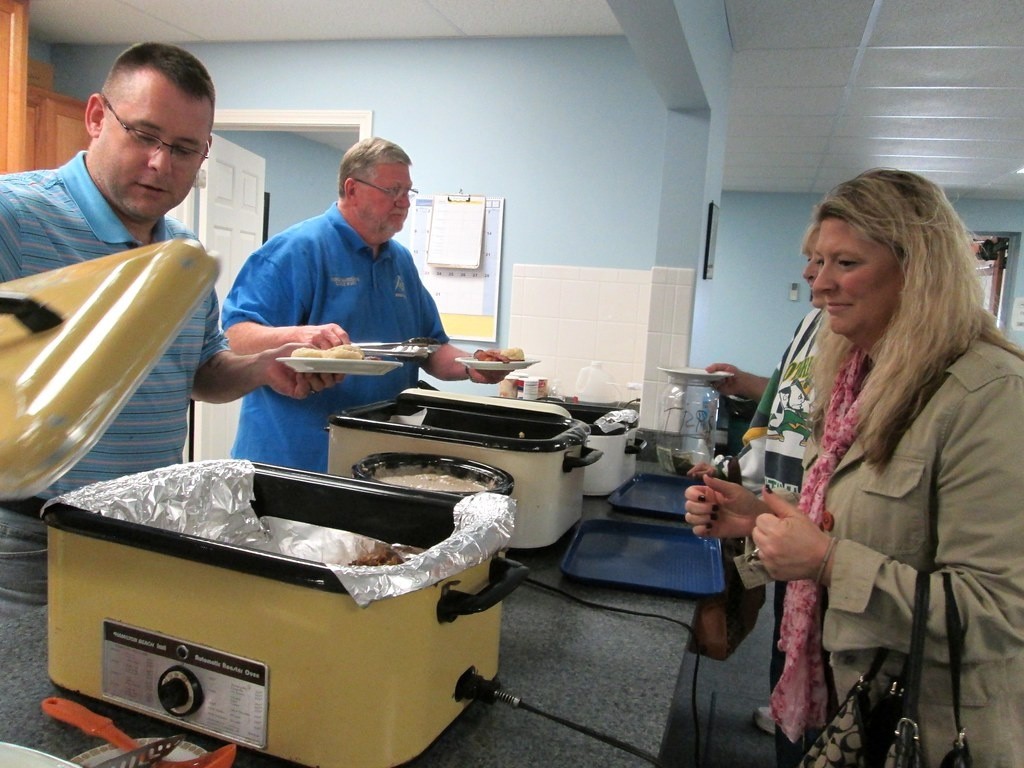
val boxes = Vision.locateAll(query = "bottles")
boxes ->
[655,375,720,475]
[548,379,566,401]
[575,359,606,402]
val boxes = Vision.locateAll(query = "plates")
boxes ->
[275,357,404,376]
[658,366,735,382]
[69,735,209,768]
[454,356,541,371]
[353,342,430,359]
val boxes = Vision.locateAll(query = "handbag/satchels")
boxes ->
[692,455,766,661]
[797,569,971,768]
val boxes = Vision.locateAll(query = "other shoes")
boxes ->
[754,706,776,733]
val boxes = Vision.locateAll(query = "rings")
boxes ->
[750,549,760,560]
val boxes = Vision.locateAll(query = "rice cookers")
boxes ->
[43,388,647,768]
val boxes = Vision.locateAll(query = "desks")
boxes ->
[0,458,701,768]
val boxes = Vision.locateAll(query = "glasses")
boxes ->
[353,177,418,201]
[104,97,210,171]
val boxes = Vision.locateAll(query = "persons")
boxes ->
[0,41,346,624]
[684,166,1024,768]
[221,137,515,473]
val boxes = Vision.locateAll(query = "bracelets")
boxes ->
[465,367,471,376]
[815,536,837,584]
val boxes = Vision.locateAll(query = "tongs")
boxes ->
[358,337,441,351]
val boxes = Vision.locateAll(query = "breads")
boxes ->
[500,347,525,361]
[290,344,365,360]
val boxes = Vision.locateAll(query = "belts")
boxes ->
[0,496,47,519]
